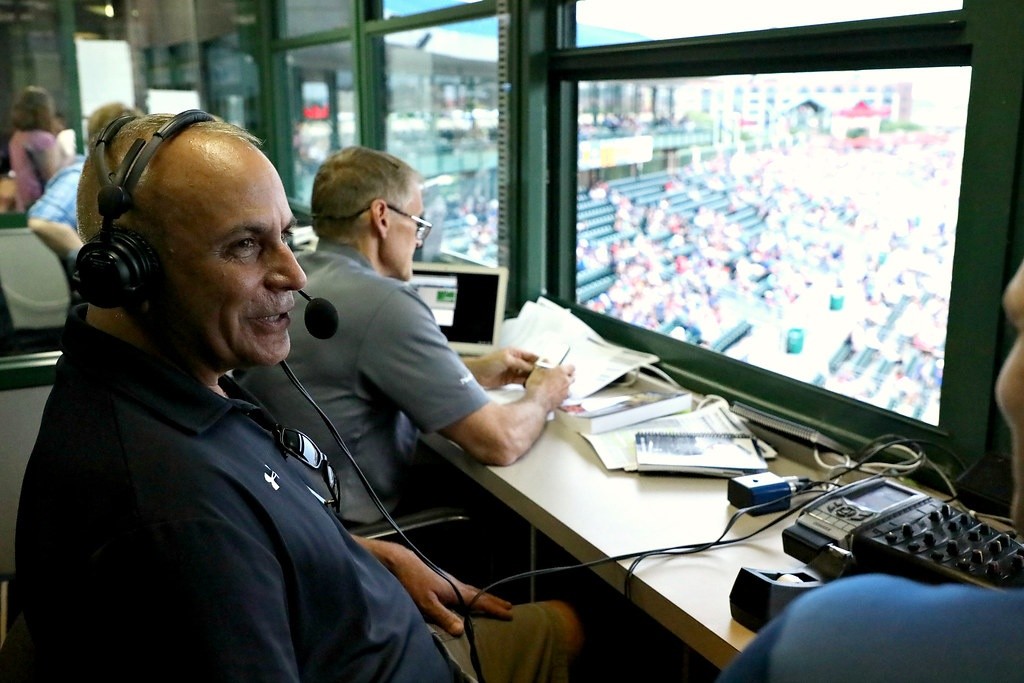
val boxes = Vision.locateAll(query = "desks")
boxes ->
[291,225,1024,683]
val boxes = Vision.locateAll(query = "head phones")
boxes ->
[68,109,215,316]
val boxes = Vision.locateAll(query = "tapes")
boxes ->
[776,574,804,583]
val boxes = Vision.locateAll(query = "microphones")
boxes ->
[297,288,339,340]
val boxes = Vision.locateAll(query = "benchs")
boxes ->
[444,144,965,426]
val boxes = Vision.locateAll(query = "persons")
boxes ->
[294,82,947,413]
[715,258,1023,683]
[7,85,146,265]
[14,109,583,683]
[234,146,568,561]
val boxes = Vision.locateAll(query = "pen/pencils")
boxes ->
[557,347,571,365]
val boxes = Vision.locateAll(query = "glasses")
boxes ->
[272,424,341,513]
[354,202,432,240]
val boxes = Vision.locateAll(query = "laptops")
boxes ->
[405,263,508,357]
